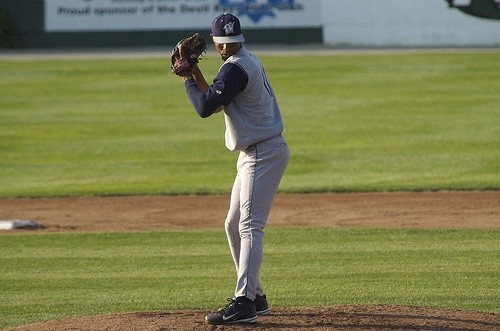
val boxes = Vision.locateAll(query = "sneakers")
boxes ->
[253,294,270,315]
[204,295,258,325]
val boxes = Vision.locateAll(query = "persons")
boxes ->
[170,14,290,325]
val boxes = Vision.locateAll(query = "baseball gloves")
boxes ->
[170,32,207,77]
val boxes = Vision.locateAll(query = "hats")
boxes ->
[211,12,245,44]
